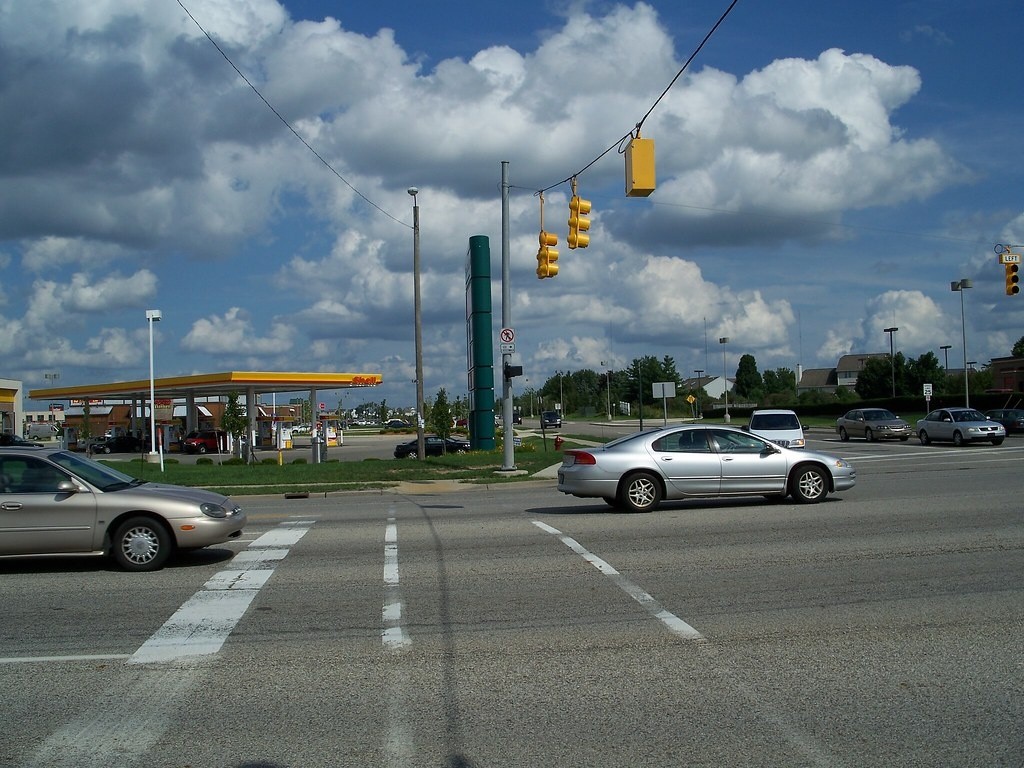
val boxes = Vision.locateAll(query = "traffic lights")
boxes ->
[535,231,558,279]
[1005,263,1019,296]
[567,196,592,250]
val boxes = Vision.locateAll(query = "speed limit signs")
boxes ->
[923,384,932,396]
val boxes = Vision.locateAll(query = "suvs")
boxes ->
[182,429,227,455]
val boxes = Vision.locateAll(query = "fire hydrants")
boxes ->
[553,435,564,452]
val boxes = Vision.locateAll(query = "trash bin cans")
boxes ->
[312,436,324,465]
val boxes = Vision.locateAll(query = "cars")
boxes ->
[0,432,45,447]
[835,408,913,442]
[394,435,471,460]
[556,424,857,513]
[77,435,111,452]
[916,407,1006,446]
[540,411,562,429]
[383,419,414,428]
[341,419,378,427]
[982,409,1024,437]
[456,418,467,429]
[0,445,248,572]
[513,410,522,425]
[292,425,312,434]
[741,410,809,451]
[91,436,147,454]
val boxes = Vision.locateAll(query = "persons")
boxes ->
[31,462,64,492]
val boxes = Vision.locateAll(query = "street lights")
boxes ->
[407,185,426,464]
[558,372,565,421]
[951,278,973,409]
[694,370,704,419]
[720,337,731,423]
[44,373,60,442]
[601,361,612,420]
[967,362,977,369]
[884,327,898,399]
[146,309,164,464]
[939,345,951,372]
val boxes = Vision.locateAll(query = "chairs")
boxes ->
[0,474,24,493]
[20,468,49,491]
[692,431,706,449]
[679,433,692,449]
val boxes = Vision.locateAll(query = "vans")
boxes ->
[985,389,1014,394]
[29,425,60,440]
